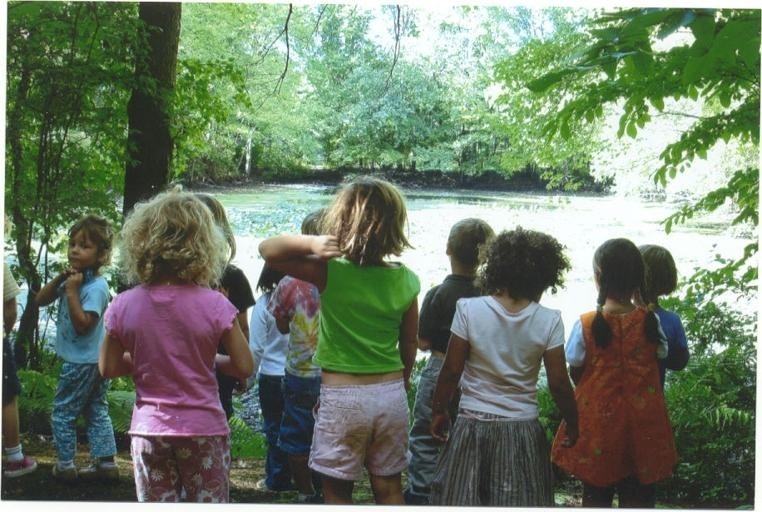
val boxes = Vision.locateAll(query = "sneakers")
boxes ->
[3,454,40,479]
[51,462,81,482]
[255,475,324,503]
[78,464,122,483]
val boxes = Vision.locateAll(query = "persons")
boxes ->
[265,208,337,504]
[34,214,120,482]
[258,175,424,504]
[194,192,257,423]
[97,191,254,504]
[3,263,38,479]
[632,243,689,391]
[549,238,679,507]
[402,218,496,505]
[429,224,580,506]
[248,262,295,491]
